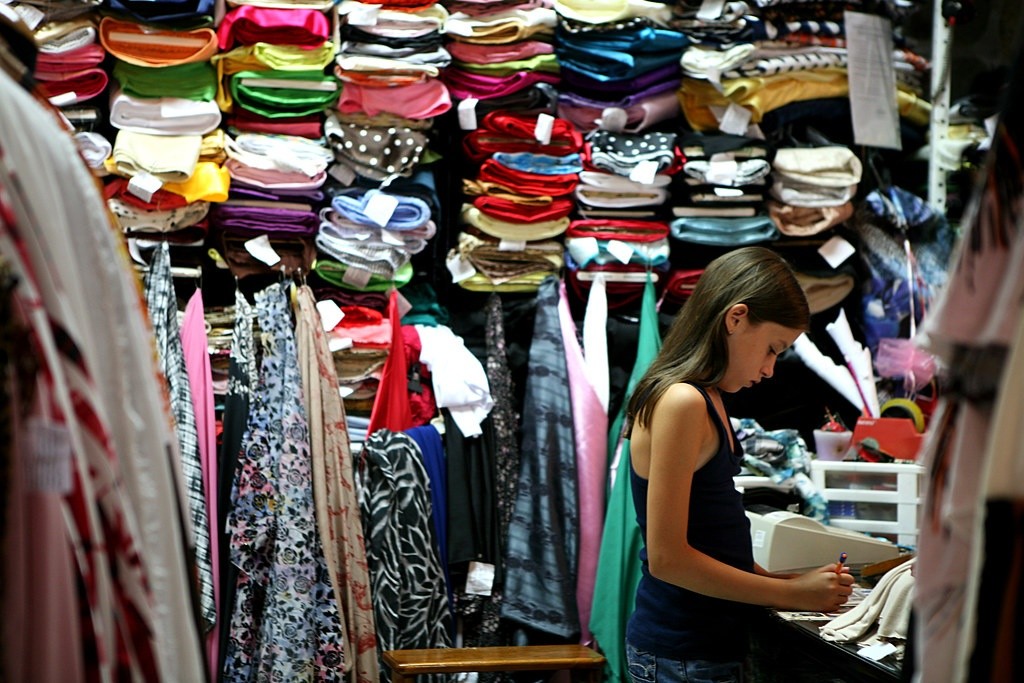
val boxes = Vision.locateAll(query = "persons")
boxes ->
[625,246,854,683]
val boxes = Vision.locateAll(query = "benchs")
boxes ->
[381,644,607,683]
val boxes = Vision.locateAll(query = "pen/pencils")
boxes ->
[835,552,846,575]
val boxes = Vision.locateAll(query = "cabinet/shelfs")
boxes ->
[809,459,928,550]
